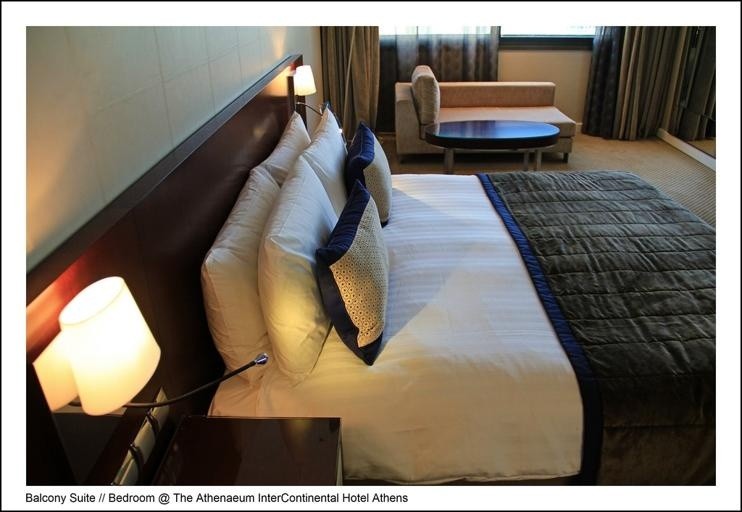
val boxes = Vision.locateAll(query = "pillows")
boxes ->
[306,101,353,217]
[341,121,392,229]
[260,109,315,179]
[316,180,388,368]
[198,161,285,387]
[410,62,441,123]
[255,152,349,390]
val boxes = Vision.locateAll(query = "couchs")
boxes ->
[392,79,577,164]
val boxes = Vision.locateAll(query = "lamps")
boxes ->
[29,273,165,423]
[288,62,316,98]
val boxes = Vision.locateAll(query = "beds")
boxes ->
[27,53,716,486]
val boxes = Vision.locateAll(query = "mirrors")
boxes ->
[652,26,716,174]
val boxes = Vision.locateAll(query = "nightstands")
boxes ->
[153,416,344,484]
